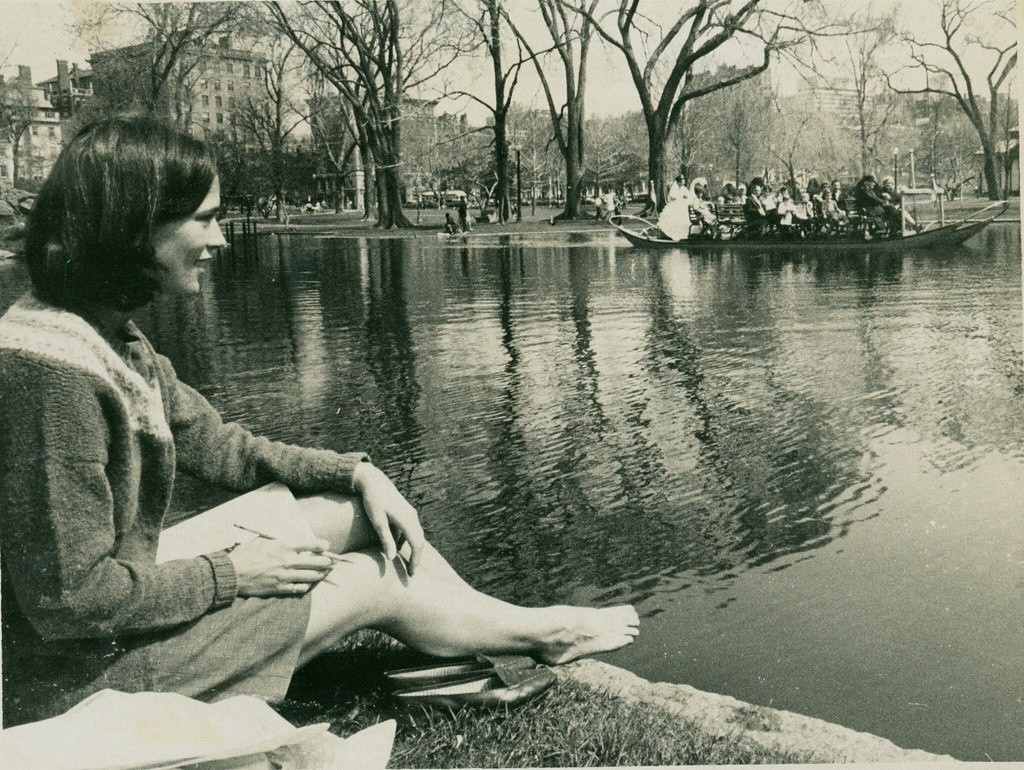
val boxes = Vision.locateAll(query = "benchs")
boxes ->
[474,209,496,224]
[694,199,889,243]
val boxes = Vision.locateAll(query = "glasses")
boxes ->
[822,186,829,188]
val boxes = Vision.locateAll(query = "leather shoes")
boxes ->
[375,651,536,716]
[385,668,557,731]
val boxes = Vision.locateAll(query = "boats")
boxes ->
[608,201,1011,249]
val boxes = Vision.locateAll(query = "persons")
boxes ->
[0,116,642,729]
[660,175,917,240]
[445,196,473,233]
[593,190,623,225]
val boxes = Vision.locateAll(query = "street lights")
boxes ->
[554,166,560,208]
[516,145,522,224]
[891,146,899,195]
[709,163,713,201]
[312,172,317,205]
